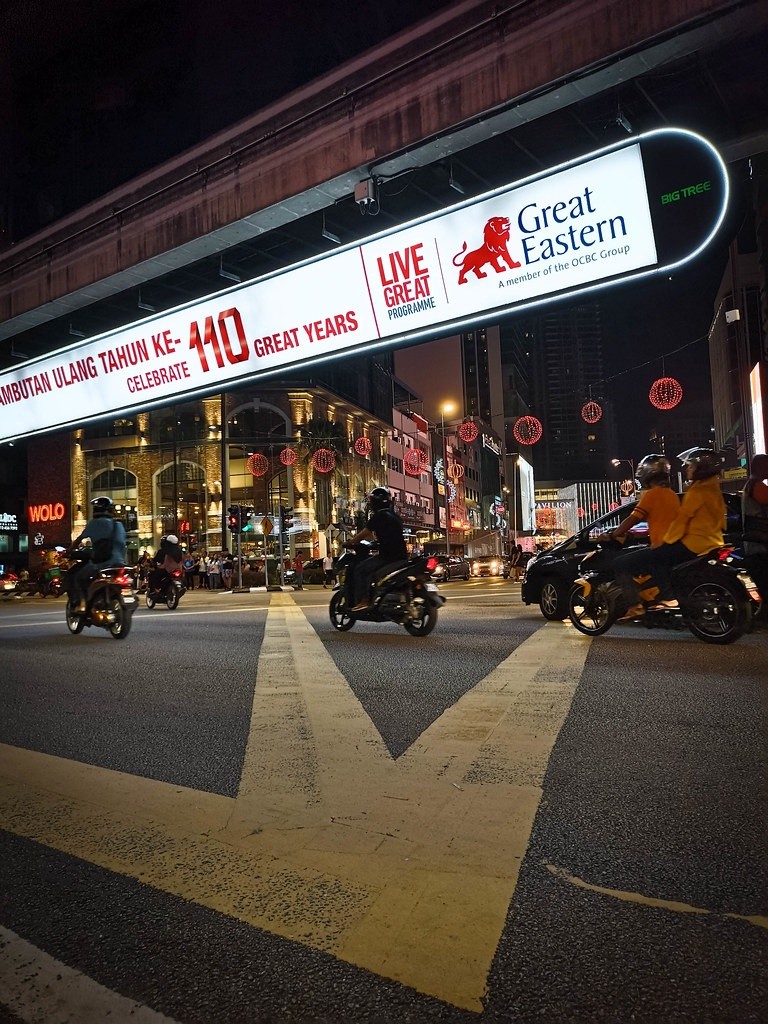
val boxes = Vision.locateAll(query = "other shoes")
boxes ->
[351,601,368,611]
[332,582,340,591]
[70,605,86,615]
[149,588,160,595]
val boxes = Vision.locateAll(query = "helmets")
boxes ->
[90,496,114,517]
[369,487,390,511]
[683,449,721,480]
[166,534,178,544]
[160,535,167,547]
[635,453,671,487]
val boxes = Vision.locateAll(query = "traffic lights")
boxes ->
[280,505,294,532]
[239,506,252,533]
[227,505,240,533]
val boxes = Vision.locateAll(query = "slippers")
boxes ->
[647,599,680,612]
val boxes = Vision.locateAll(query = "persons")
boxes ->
[148,534,182,595]
[597,453,682,622]
[323,552,335,588]
[342,488,410,611]
[514,544,523,583]
[295,551,304,590]
[509,540,518,579]
[741,455,768,556]
[648,450,728,612]
[183,547,265,592]
[64,496,127,612]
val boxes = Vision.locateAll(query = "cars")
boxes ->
[473,555,508,576]
[0,574,17,591]
[504,551,536,579]
[424,555,471,582]
[521,492,742,620]
[244,557,274,585]
[280,557,340,585]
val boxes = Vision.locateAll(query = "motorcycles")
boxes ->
[145,558,187,610]
[565,526,761,644]
[37,576,62,598]
[61,545,140,639]
[328,539,447,637]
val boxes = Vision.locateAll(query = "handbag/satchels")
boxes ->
[743,514,768,544]
[510,567,516,578]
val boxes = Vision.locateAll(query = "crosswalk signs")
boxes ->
[260,516,273,537]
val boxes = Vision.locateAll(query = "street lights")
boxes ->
[612,459,636,497]
[442,404,453,554]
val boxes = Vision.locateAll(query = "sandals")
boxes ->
[617,606,645,621]
